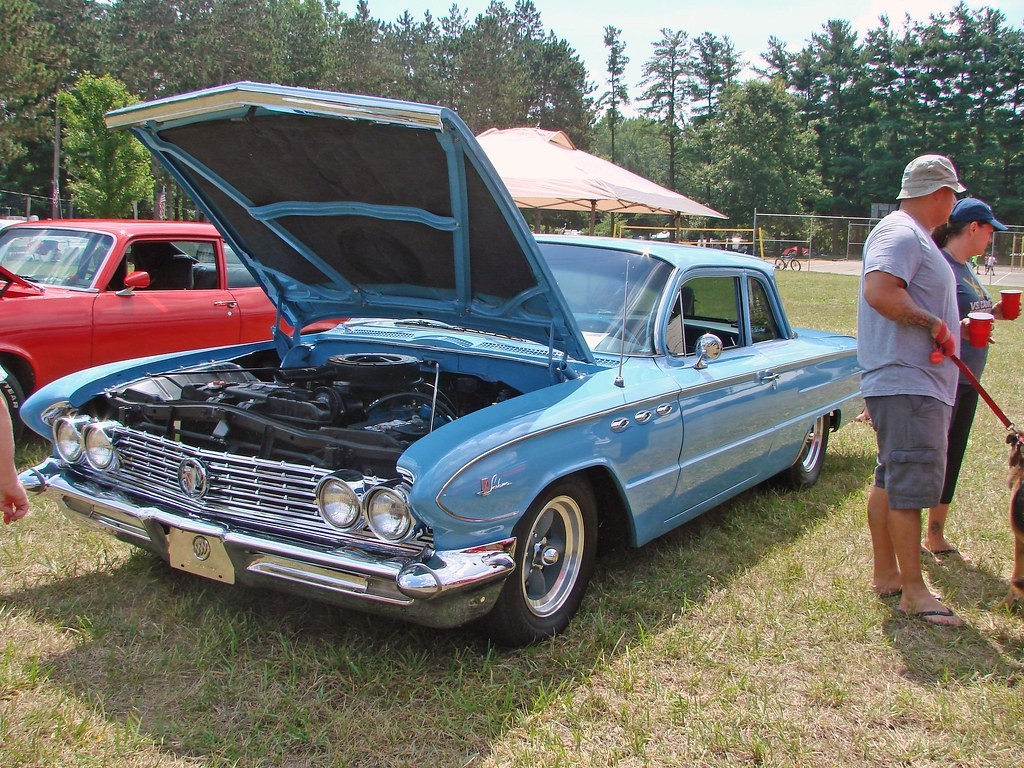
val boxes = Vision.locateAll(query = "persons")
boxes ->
[856,154,1022,629]
[697,232,707,248]
[0,366,29,524]
[731,233,743,252]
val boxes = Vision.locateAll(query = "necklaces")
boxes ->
[945,246,961,262]
[903,210,917,221]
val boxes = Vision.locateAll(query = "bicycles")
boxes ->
[775,253,802,271]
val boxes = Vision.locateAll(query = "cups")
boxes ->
[999,290,1022,321]
[967,312,994,348]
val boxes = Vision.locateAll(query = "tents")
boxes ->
[475,128,730,243]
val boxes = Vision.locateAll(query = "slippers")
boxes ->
[897,597,964,627]
[920,537,971,565]
[876,589,941,602]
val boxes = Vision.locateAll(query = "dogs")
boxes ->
[999,430,1024,612]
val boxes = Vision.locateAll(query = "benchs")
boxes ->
[194,266,254,289]
[604,315,738,354]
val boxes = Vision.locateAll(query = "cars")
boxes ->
[0,218,352,448]
[17,80,861,667]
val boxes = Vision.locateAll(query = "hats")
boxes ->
[895,155,966,199]
[950,196,1008,230]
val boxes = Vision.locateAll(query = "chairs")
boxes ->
[100,250,127,291]
